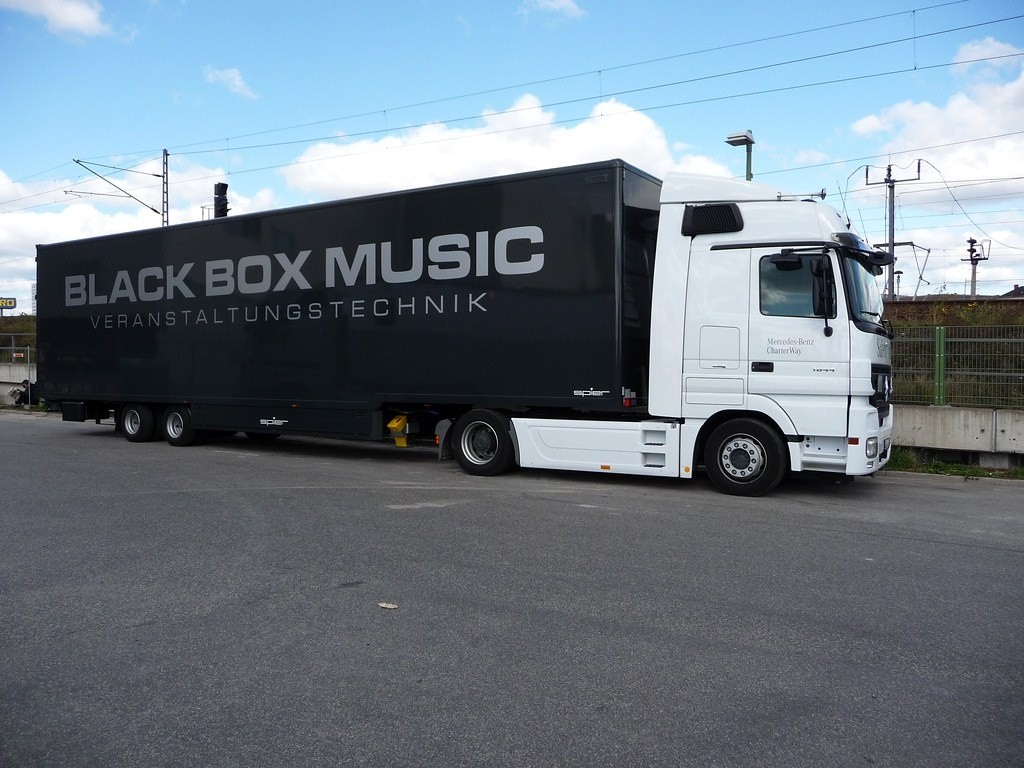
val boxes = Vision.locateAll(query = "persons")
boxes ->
[15,379,39,407]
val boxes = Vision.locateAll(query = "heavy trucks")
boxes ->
[33,157,903,495]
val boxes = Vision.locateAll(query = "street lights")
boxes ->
[725,128,755,183]
